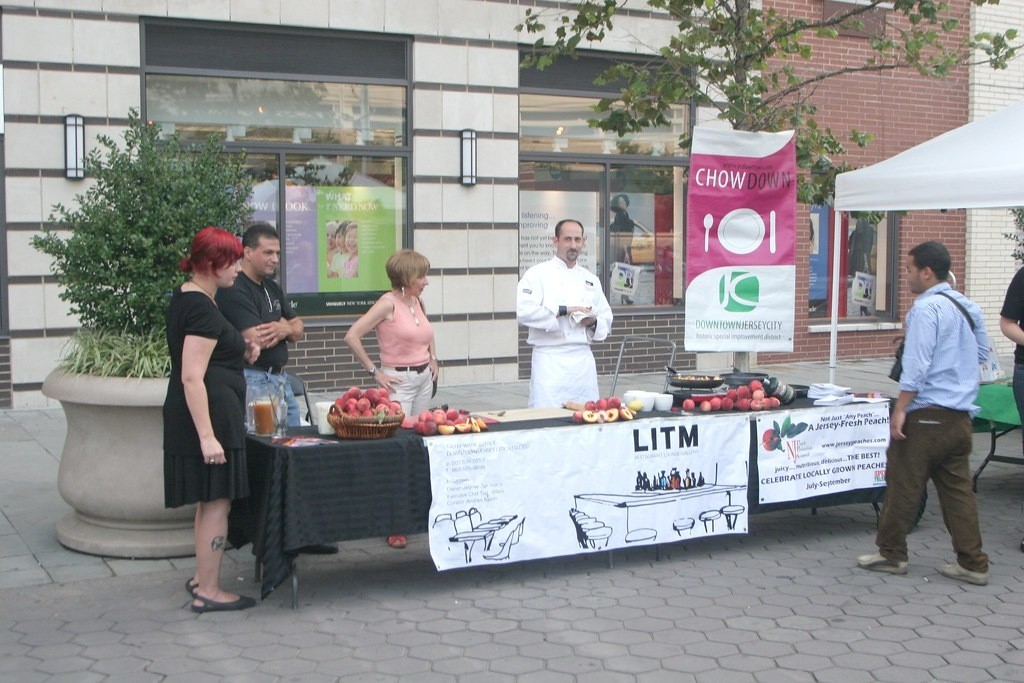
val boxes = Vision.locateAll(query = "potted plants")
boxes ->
[29,107,265,558]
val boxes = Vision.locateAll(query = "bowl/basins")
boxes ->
[624,390,673,412]
[788,384,809,396]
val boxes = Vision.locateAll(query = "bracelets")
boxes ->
[430,355,438,361]
[368,367,377,376]
[558,306,567,316]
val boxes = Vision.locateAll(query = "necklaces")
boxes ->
[189,278,230,326]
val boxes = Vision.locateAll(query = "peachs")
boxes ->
[683,399,695,411]
[701,380,780,411]
[573,396,636,422]
[334,387,403,418]
[414,408,488,433]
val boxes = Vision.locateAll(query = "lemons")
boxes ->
[628,401,644,411]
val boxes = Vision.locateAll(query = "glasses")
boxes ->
[409,305,419,327]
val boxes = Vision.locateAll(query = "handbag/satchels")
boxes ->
[888,333,904,383]
[431,370,437,399]
[610,261,640,296]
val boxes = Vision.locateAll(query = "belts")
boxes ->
[395,363,429,373]
[243,364,283,375]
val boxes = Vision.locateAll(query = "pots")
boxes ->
[664,365,724,388]
[719,367,768,385]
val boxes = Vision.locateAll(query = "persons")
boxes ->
[810,218,815,253]
[610,194,653,305]
[856,240,992,587]
[1000,265,1024,554]
[515,219,615,410]
[848,219,874,317]
[343,249,439,549]
[213,223,340,556]
[326,220,358,278]
[162,225,257,613]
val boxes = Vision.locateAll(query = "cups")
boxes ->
[315,402,336,434]
[270,405,288,439]
[252,396,279,436]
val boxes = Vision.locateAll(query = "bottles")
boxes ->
[561,402,585,411]
[759,377,797,404]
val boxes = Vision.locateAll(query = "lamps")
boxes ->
[651,143,660,157]
[290,128,302,143]
[354,129,366,146]
[64,113,87,180]
[601,140,612,154]
[552,137,562,152]
[459,128,479,187]
[224,125,234,142]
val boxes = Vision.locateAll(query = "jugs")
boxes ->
[245,375,275,434]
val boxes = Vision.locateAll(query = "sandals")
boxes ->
[387,536,407,548]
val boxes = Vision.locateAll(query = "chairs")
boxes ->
[285,372,313,426]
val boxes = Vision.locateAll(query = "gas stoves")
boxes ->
[667,387,727,402]
[729,387,737,390]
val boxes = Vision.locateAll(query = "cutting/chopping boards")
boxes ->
[476,408,580,423]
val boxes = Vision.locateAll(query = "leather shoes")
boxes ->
[296,543,339,554]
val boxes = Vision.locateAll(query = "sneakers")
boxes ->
[942,562,988,585]
[857,551,907,574]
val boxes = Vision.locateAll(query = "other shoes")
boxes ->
[186,577,199,596]
[190,595,256,613]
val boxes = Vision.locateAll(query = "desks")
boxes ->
[972,376,1024,493]
[226,385,898,609]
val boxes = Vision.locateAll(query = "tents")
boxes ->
[828,105,1024,388]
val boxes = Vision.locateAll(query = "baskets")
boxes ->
[328,401,404,440]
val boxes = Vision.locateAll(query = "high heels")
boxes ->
[621,295,633,304]
